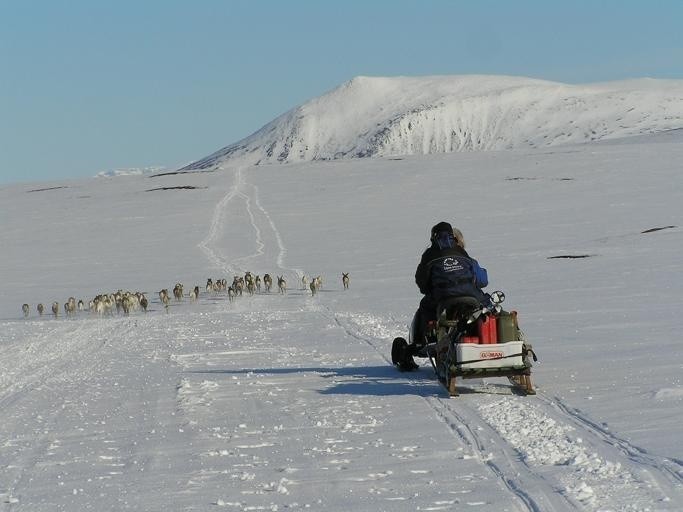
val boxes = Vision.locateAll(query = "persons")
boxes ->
[452,228,464,249]
[407,221,491,346]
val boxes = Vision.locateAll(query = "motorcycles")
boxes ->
[391,293,536,398]
